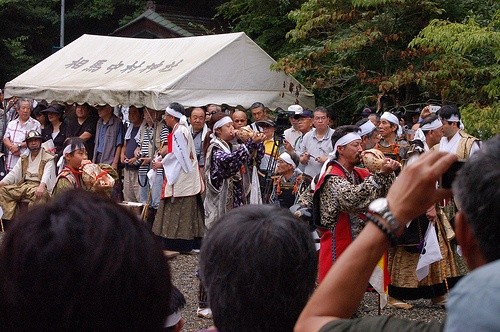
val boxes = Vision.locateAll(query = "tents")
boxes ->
[2,33,316,168]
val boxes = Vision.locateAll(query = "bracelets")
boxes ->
[365,213,397,246]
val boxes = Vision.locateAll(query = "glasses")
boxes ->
[208,111,216,115]
[234,119,246,124]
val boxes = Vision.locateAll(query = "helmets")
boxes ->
[23,129,43,142]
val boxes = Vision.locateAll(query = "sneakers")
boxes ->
[196,305,213,320]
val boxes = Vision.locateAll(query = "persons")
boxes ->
[0,87,481,318]
[199,205,317,332]
[296,132,500,332]
[166,284,186,332]
[0,189,172,332]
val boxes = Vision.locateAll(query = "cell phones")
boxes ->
[438,158,465,190]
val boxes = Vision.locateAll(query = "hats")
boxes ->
[256,117,276,127]
[294,110,313,121]
[41,104,61,114]
[288,104,303,116]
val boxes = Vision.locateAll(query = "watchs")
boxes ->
[18,143,21,148]
[369,197,402,235]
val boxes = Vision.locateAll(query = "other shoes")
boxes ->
[388,297,411,309]
[432,296,448,306]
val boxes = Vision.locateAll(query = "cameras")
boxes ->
[273,110,293,135]
[133,153,147,168]
